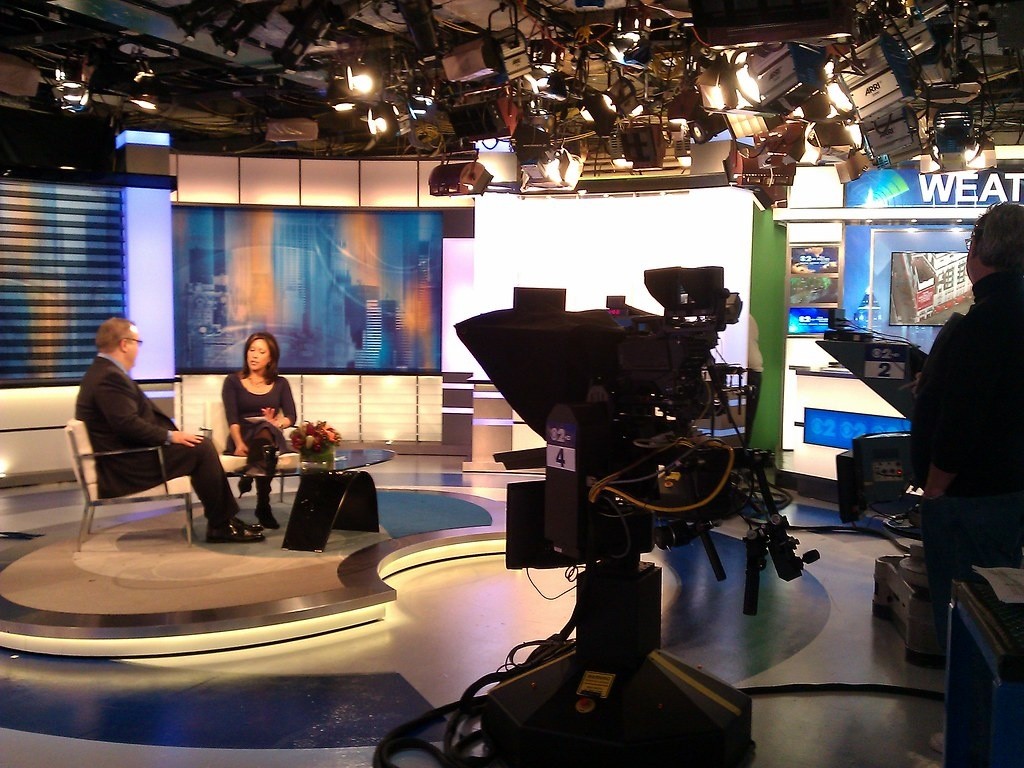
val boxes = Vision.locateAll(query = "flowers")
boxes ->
[289,420,342,452]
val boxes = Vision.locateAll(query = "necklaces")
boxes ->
[248,376,264,385]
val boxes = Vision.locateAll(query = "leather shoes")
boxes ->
[206,522,265,542]
[231,516,264,531]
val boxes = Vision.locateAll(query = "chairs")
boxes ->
[206,402,301,504]
[65,418,193,551]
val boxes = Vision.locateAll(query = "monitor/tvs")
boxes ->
[889,251,975,327]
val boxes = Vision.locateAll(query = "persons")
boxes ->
[75,318,268,541]
[809,284,838,302]
[911,203,1024,751]
[222,331,298,528]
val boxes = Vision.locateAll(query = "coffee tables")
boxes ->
[235,448,395,553]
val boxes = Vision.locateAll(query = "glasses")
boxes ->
[118,338,143,347]
[965,239,973,251]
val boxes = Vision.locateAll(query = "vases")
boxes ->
[299,444,336,469]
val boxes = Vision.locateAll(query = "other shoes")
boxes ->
[928,733,944,754]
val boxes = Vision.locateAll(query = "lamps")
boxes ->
[30,0,1024,210]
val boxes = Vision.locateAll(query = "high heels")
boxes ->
[255,509,280,529]
[238,479,252,499]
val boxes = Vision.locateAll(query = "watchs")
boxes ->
[168,429,172,437]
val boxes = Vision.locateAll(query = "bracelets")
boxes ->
[275,419,284,429]
